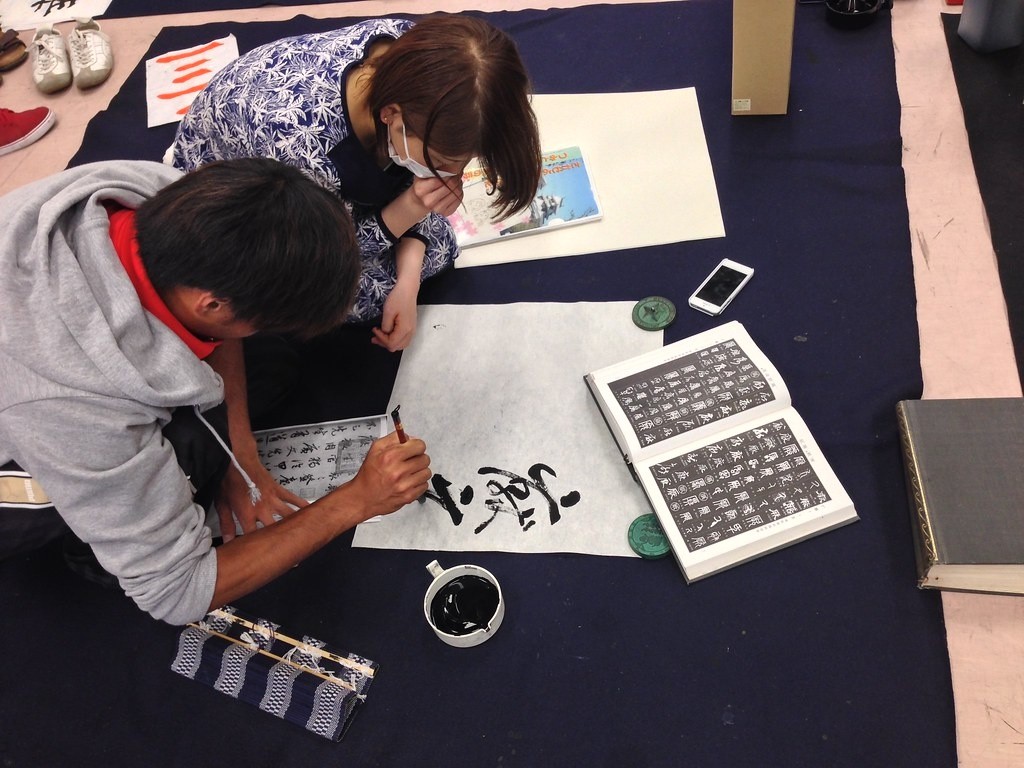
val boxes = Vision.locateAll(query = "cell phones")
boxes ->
[688,258,755,317]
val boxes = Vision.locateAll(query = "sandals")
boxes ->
[0,25,27,71]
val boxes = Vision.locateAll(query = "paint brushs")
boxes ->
[390,405,424,506]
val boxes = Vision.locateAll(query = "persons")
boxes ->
[1,161,431,625]
[161,15,542,354]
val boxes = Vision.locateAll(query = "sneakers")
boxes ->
[68,15,115,89]
[0,106,54,156]
[26,20,71,93]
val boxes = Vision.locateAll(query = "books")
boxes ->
[583,318,861,587]
[896,396,1024,598]
[446,145,604,249]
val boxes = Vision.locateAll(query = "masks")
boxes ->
[386,122,458,179]
